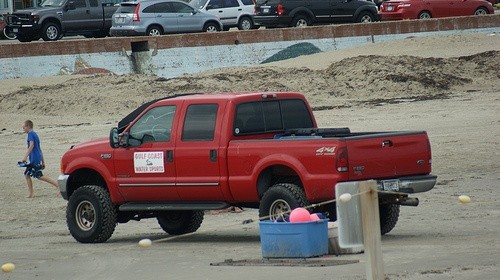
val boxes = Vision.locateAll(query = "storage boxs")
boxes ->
[259,219,330,259]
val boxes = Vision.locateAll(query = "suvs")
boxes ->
[253,0,381,29]
[185,0,257,30]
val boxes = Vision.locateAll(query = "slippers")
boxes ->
[17,161,29,167]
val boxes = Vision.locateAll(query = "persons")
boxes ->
[22,119,59,198]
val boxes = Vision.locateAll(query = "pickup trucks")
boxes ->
[7,0,120,42]
[56,90,437,244]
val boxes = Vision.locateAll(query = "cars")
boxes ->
[109,0,224,37]
[379,0,495,22]
